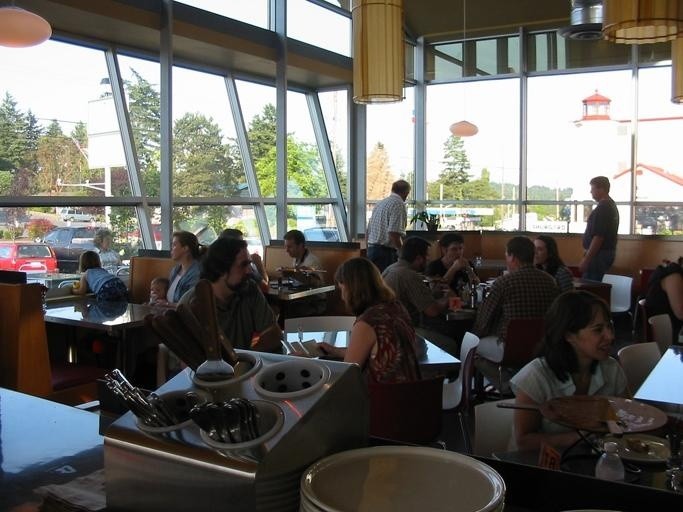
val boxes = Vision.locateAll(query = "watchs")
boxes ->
[466,265,474,276]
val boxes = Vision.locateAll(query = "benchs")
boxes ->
[1,285,99,411]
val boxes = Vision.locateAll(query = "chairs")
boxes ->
[444,332,479,452]
[639,268,656,295]
[648,315,674,353]
[601,274,632,322]
[365,377,444,450]
[617,342,663,395]
[663,274,683,322]
[475,318,549,401]
[610,298,648,359]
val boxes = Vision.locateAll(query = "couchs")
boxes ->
[407,228,480,262]
[127,254,182,306]
[266,241,362,313]
[481,232,683,299]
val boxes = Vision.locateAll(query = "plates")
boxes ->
[541,396,667,432]
[301,446,506,512]
[598,434,671,462]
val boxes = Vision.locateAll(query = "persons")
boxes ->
[508,290,634,452]
[156,232,205,308]
[382,237,460,359]
[642,253,683,351]
[426,234,479,298]
[534,235,574,297]
[142,278,168,308]
[175,237,284,373]
[577,176,620,281]
[289,257,428,384]
[364,179,412,273]
[73,252,127,303]
[276,228,328,329]
[219,228,270,294]
[472,236,559,396]
[92,228,122,274]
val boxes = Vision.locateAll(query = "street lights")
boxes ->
[99,77,117,224]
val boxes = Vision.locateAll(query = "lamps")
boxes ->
[0,1,51,49]
[351,2,403,104]
[602,0,683,44]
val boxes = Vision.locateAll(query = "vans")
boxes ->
[0,242,57,283]
[60,209,96,222]
[304,226,341,242]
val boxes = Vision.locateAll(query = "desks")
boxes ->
[633,347,683,418]
[369,398,683,496]
[283,315,461,380]
[264,280,336,329]
[448,296,486,319]
[44,300,171,386]
[474,258,510,283]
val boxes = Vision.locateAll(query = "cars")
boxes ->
[113,222,162,242]
[44,227,132,273]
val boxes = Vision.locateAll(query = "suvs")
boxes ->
[24,219,58,230]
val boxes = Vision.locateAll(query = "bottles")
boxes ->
[594,442,624,482]
[468,267,476,286]
[470,283,477,308]
